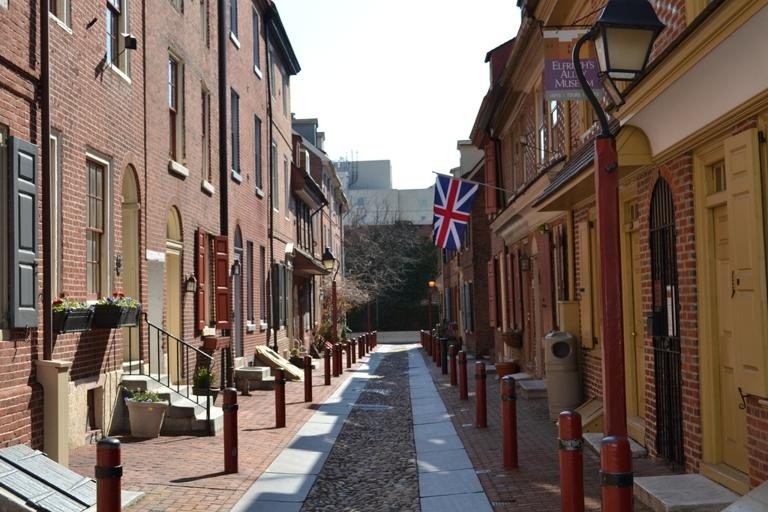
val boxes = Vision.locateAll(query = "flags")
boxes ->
[430,172,481,253]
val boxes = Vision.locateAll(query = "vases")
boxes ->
[495,361,520,379]
[125,400,169,438]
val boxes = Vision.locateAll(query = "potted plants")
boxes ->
[191,365,221,396]
[501,327,522,348]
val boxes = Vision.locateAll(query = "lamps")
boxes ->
[518,255,531,270]
[232,259,241,275]
[186,273,198,293]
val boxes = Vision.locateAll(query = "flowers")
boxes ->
[96,292,140,307]
[51,290,88,310]
[120,386,161,406]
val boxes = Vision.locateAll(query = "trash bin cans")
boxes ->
[544,330,584,423]
